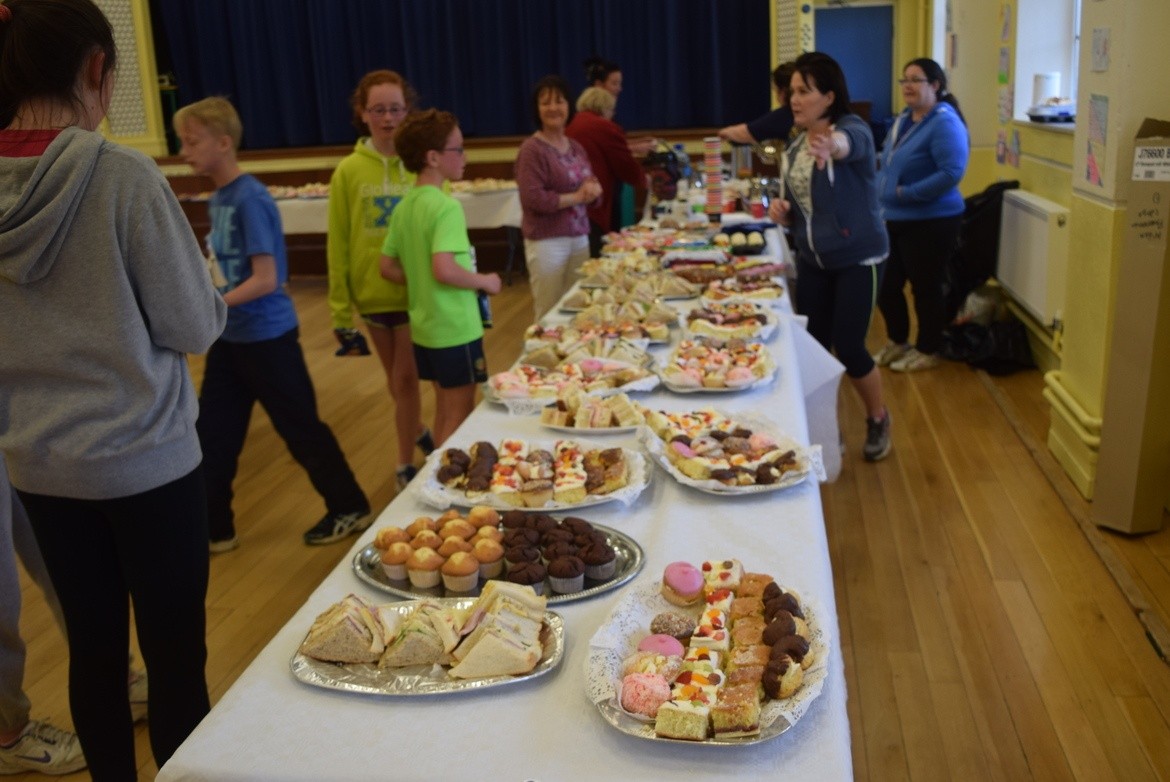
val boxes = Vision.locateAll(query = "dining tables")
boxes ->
[152,177,851,782]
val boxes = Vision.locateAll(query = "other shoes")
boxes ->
[397,465,416,490]
[416,427,435,457]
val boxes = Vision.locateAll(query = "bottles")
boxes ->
[749,178,765,218]
[673,145,691,178]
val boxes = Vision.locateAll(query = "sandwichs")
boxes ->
[301,580,547,680]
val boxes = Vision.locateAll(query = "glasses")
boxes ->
[898,75,927,87]
[365,100,407,117]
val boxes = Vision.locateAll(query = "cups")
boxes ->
[703,136,723,223]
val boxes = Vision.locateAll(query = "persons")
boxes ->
[515,74,604,324]
[0,0,229,782]
[0,449,151,776]
[715,61,799,146]
[564,84,649,261]
[766,51,894,464]
[323,70,453,491]
[171,98,373,553]
[872,56,973,374]
[379,108,504,447]
[585,59,624,97]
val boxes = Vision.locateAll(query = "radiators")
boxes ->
[995,190,1070,327]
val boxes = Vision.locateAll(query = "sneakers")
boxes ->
[889,347,937,373]
[206,516,238,553]
[863,407,890,461]
[0,717,88,776]
[870,342,909,367]
[127,664,148,723]
[304,509,373,546]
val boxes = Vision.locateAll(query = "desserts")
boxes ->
[613,560,815,741]
[487,323,656,398]
[644,405,803,487]
[437,437,629,509]
[539,383,648,428]
[663,334,764,388]
[374,503,617,595]
[563,217,785,338]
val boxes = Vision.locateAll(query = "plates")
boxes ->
[1026,113,1075,123]
[290,226,826,748]
